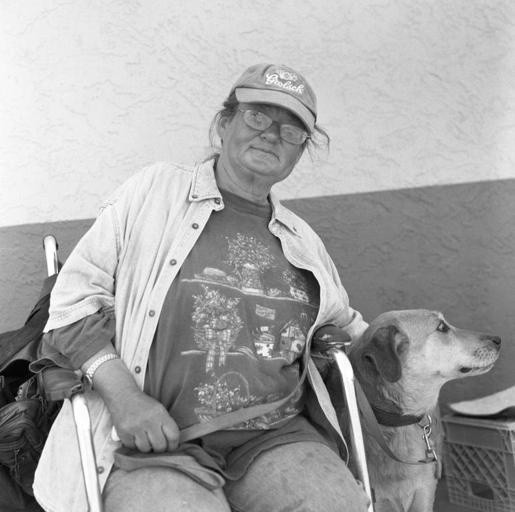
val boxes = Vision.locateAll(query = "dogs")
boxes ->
[346,308,501,512]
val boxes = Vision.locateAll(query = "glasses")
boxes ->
[236,107,308,145]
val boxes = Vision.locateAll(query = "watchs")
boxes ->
[82,353,121,392]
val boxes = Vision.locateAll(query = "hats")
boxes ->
[228,61,320,133]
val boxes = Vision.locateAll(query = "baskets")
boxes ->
[442,414,514,510]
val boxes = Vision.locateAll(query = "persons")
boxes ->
[29,62,373,512]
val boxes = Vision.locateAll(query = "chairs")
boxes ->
[44,232,373,512]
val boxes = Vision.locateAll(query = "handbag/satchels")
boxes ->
[0,398,61,498]
